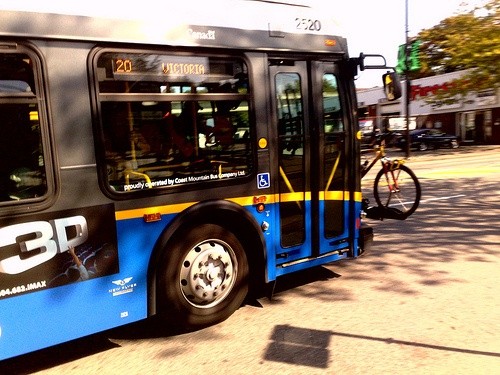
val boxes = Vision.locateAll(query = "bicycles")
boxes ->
[359,132,421,221]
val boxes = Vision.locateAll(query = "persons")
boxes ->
[381,124,390,133]
[111,101,243,169]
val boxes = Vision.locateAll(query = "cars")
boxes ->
[359,129,461,149]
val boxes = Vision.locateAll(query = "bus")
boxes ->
[0,0,402,359]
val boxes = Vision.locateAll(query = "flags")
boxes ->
[397,43,409,72]
[409,40,422,70]
